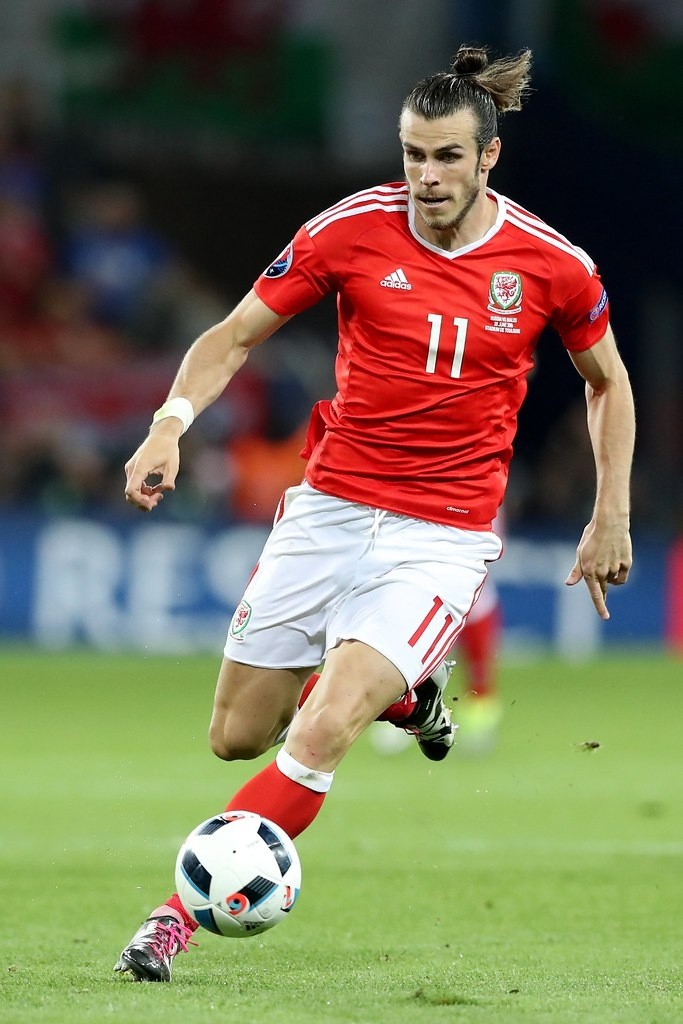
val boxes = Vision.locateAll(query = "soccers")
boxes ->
[174,808,305,941]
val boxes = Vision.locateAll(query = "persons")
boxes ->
[113,44,635,981]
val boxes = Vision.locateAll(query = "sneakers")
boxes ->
[113,915,198,982]
[389,659,459,762]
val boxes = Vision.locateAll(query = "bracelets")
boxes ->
[150,398,195,438]
